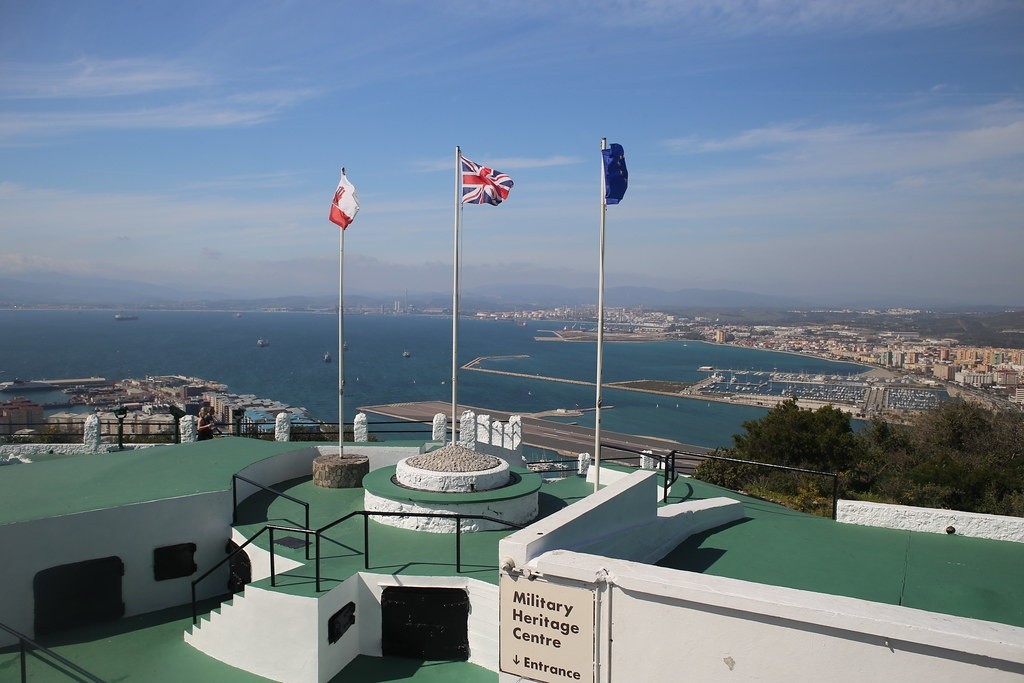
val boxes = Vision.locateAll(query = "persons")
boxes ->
[196,407,215,441]
[204,406,223,438]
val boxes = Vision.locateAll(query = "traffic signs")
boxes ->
[494,572,596,683]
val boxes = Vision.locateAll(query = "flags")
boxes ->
[600,142,629,206]
[329,173,360,229]
[458,154,515,207]
